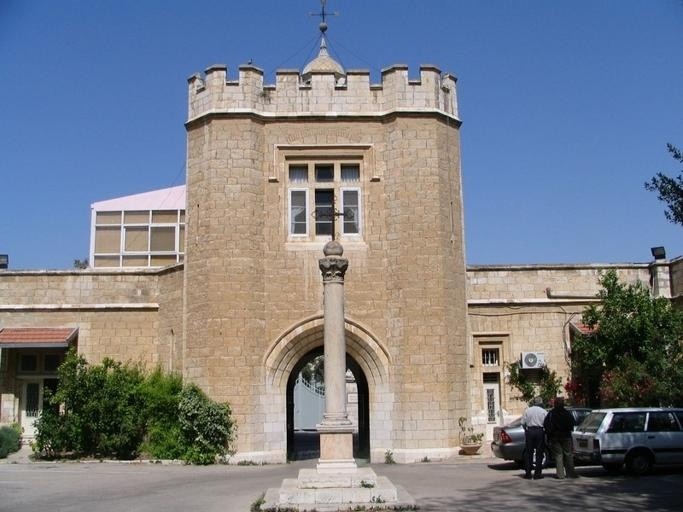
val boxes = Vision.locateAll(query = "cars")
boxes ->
[491,406,594,469]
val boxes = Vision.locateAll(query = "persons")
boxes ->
[520,397,548,480]
[543,398,580,479]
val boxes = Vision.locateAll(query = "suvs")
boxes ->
[573,406,682,475]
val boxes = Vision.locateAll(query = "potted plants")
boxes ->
[456,414,485,455]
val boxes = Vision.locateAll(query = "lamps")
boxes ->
[0,254,8,269]
[650,246,666,260]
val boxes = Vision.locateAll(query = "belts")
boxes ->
[527,426,542,431]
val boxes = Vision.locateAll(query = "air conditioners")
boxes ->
[518,351,545,370]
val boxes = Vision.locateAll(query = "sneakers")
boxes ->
[524,473,543,481]
